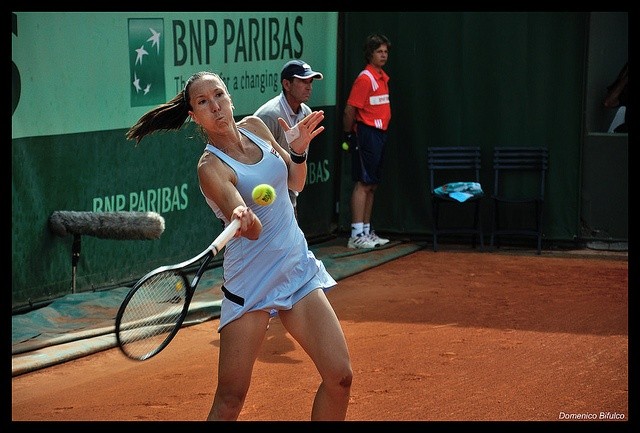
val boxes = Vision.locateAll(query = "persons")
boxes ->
[343,33,391,249]
[126,71,352,421]
[253,59,323,223]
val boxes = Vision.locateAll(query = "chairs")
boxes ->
[488,146,549,256]
[426,146,486,253]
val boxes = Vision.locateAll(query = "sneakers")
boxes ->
[348,234,380,249]
[367,230,390,245]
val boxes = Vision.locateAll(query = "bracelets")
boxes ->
[288,145,306,157]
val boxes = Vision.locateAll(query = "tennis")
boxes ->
[251,183,276,207]
[342,143,349,150]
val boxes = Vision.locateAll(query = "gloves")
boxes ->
[339,131,351,146]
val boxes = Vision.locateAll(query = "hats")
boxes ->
[281,60,323,80]
